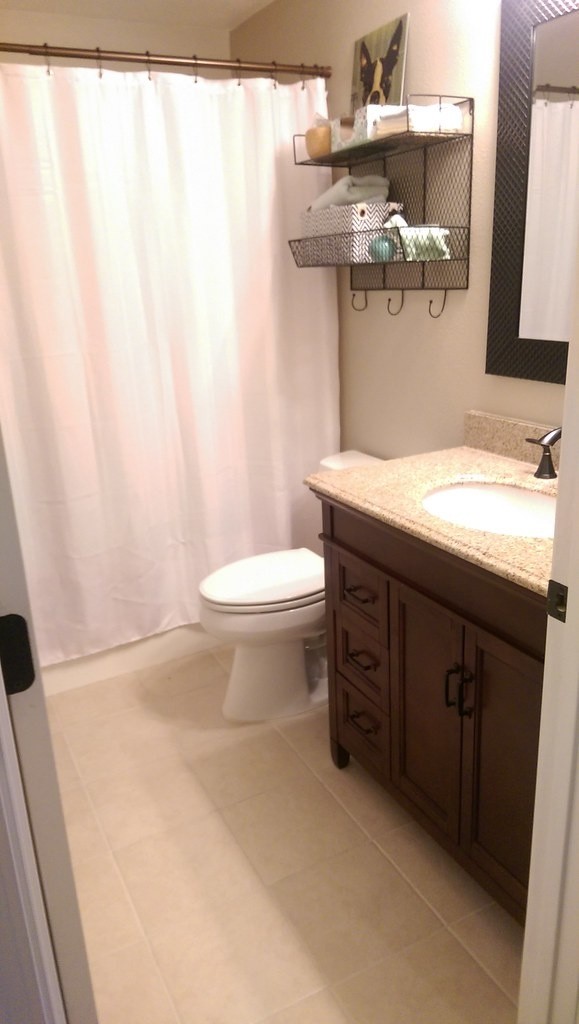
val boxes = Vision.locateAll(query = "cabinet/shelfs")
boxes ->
[388,538,548,926]
[288,92,474,319]
[310,490,390,791]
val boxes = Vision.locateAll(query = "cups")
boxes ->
[305,127,331,158]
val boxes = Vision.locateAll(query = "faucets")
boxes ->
[538,427,561,447]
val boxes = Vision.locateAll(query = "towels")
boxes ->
[304,175,390,213]
[376,102,463,136]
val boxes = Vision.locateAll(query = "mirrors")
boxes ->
[483,1,579,384]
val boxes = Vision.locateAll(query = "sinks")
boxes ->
[420,478,557,541]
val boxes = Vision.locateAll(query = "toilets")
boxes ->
[196,447,389,723]
